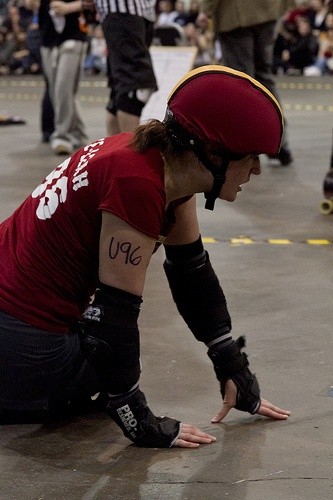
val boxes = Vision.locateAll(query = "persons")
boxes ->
[0,0,333,214]
[0,65,290,448]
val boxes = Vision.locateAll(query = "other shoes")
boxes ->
[304,66,322,78]
[41,131,80,154]
[268,148,292,166]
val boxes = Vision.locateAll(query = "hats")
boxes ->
[168,64,285,155]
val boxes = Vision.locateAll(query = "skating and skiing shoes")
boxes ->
[319,155,333,215]
